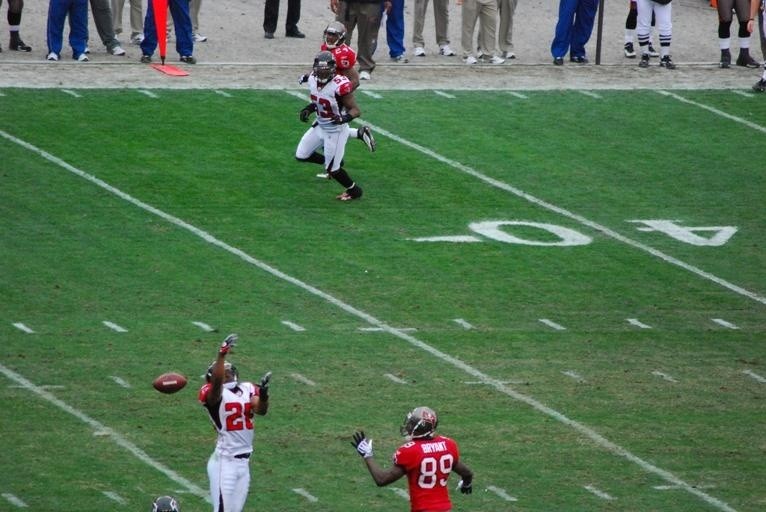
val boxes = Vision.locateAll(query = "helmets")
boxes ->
[324,21,348,49]
[313,51,337,82]
[400,407,437,441]
[207,360,238,383]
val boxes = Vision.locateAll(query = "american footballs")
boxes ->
[152,372,187,394]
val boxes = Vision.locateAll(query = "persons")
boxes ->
[6,2,208,65]
[263,0,306,38]
[350,407,474,511]
[551,1,599,65]
[299,21,376,152]
[624,1,676,69]
[330,1,517,80]
[716,0,766,92]
[198,333,273,512]
[295,51,363,201]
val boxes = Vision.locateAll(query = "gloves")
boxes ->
[329,113,343,125]
[300,109,310,121]
[299,73,308,84]
[255,372,272,395]
[219,334,237,354]
[351,431,373,459]
[456,480,472,494]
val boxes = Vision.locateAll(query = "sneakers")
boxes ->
[9,31,207,63]
[317,173,333,178]
[336,187,362,201]
[286,31,304,38]
[359,127,376,152]
[265,30,274,39]
